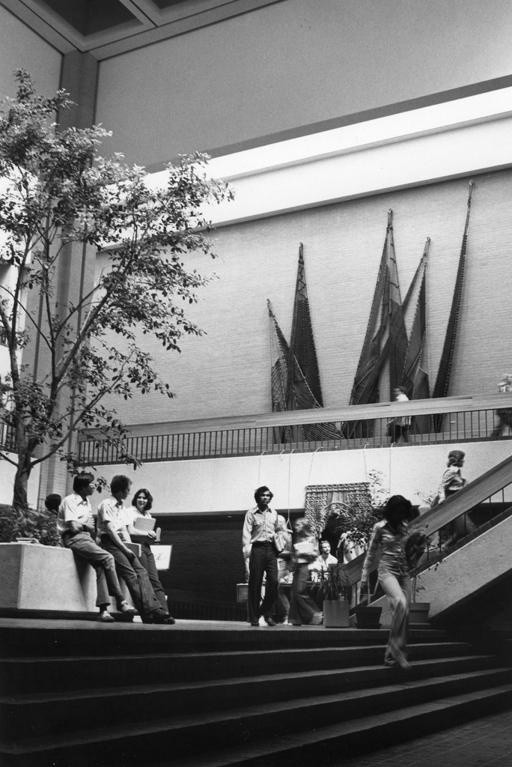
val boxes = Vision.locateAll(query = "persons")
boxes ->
[56,473,134,622]
[127,489,175,623]
[439,449,471,547]
[97,475,176,624]
[276,514,289,625]
[45,494,60,514]
[390,387,411,445]
[289,518,323,626]
[320,540,338,576]
[493,373,512,438]
[360,495,428,670]
[243,486,286,626]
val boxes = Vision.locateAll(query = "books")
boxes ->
[275,531,286,552]
[294,541,315,563]
[135,517,155,531]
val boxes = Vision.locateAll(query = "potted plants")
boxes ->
[309,565,349,628]
[315,469,392,628]
[404,526,450,629]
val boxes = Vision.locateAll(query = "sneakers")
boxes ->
[383,657,397,666]
[100,609,116,621]
[118,599,139,614]
[399,657,411,671]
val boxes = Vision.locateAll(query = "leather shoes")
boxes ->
[264,615,276,625]
[143,615,174,624]
[251,616,259,626]
[144,607,169,616]
